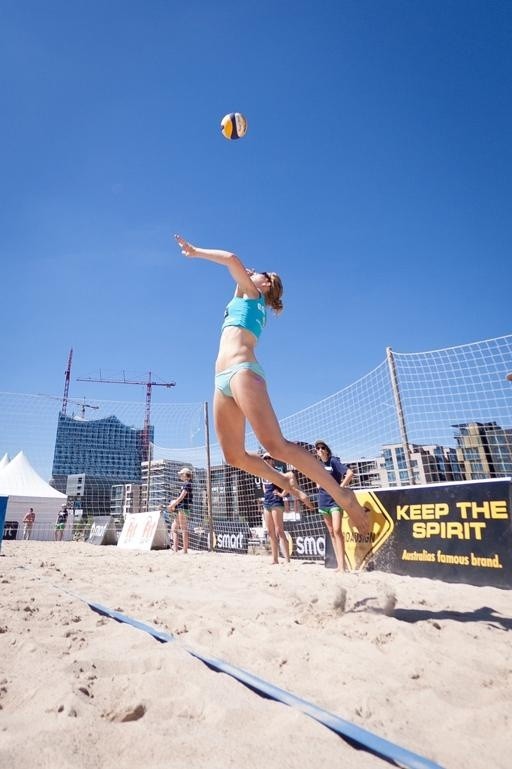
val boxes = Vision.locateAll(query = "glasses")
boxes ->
[264,457,271,459]
[260,272,271,282]
[316,446,326,450]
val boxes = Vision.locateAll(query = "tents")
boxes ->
[1,449,69,542]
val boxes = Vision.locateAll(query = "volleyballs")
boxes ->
[220,113,248,140]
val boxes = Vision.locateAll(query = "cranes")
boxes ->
[36,347,99,419]
[75,370,176,460]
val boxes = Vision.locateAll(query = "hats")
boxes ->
[262,452,272,459]
[178,468,192,475]
[313,439,328,447]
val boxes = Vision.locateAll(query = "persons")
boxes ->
[313,439,355,574]
[257,452,294,566]
[165,466,195,555]
[21,508,36,540]
[171,231,374,538]
[54,504,69,542]
